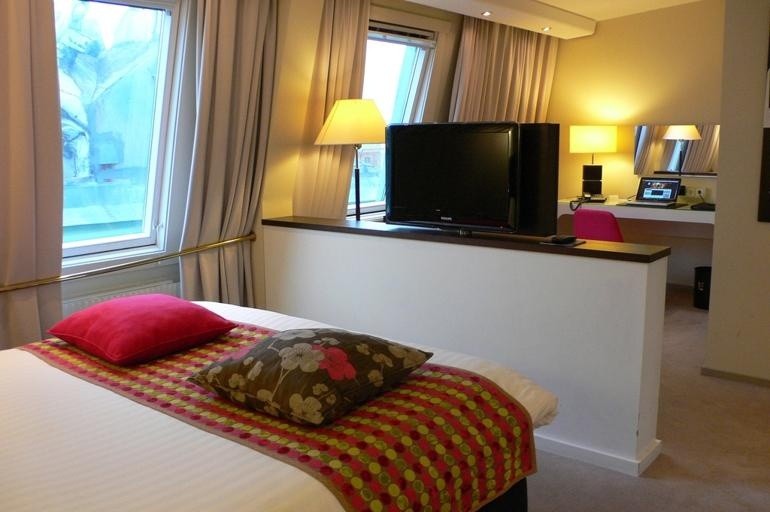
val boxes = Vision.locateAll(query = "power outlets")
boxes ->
[695,186,706,198]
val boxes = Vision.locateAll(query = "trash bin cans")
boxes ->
[694,266,711,310]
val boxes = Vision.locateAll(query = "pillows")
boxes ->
[47,293,234,360]
[187,327,432,423]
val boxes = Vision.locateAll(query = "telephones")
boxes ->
[582,192,607,203]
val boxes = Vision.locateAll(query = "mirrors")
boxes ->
[633,124,720,176]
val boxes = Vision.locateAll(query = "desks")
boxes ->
[555,197,715,240]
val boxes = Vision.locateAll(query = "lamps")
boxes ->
[663,125,702,175]
[314,100,389,220]
[567,127,619,194]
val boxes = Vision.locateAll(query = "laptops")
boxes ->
[627,177,681,208]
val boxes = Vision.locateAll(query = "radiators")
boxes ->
[63,281,182,321]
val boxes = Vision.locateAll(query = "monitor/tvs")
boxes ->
[385,122,521,237]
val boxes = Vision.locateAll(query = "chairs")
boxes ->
[574,208,624,244]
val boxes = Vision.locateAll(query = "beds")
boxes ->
[0,302,559,512]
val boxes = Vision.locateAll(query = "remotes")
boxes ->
[551,235,578,244]
